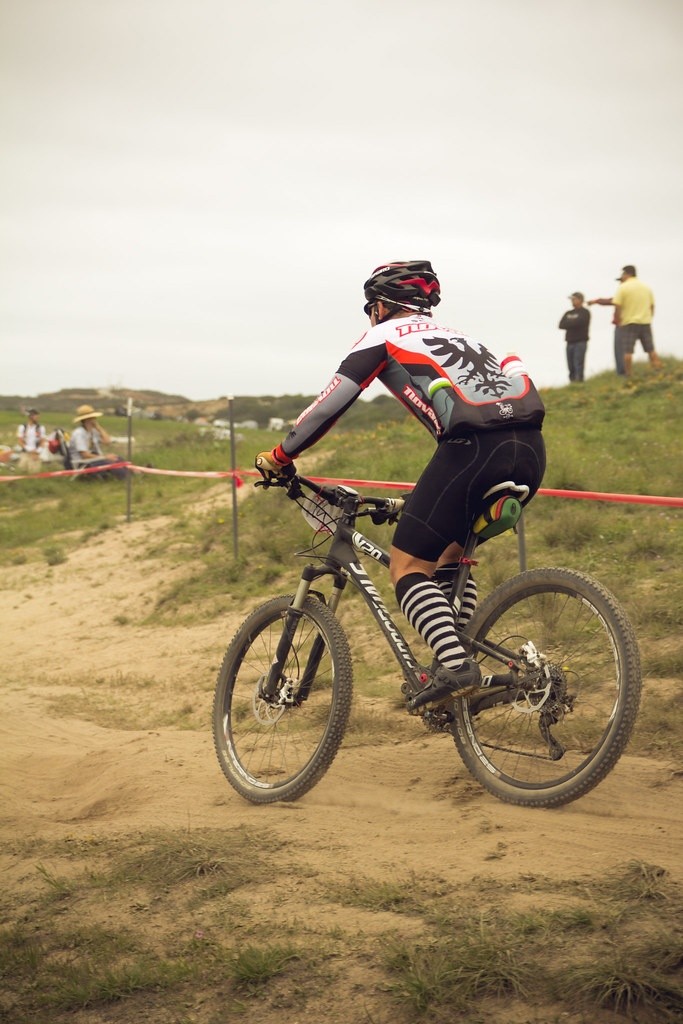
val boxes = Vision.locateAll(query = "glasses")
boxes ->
[364,300,385,316]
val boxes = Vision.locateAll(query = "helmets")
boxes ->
[364,260,441,313]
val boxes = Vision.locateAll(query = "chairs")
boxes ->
[56,429,117,486]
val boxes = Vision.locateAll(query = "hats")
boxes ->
[25,409,40,417]
[73,404,103,423]
[567,292,583,300]
[616,277,623,281]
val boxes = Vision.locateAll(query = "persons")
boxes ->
[559,292,590,383]
[19,410,46,460]
[68,405,134,480]
[587,265,665,378]
[256,260,546,713]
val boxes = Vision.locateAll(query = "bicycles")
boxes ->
[209,456,644,809]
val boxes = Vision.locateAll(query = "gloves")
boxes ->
[255,449,297,490]
[399,493,410,513]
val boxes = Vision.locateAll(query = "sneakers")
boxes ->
[406,654,481,716]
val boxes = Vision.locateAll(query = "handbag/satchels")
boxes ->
[63,455,72,470]
